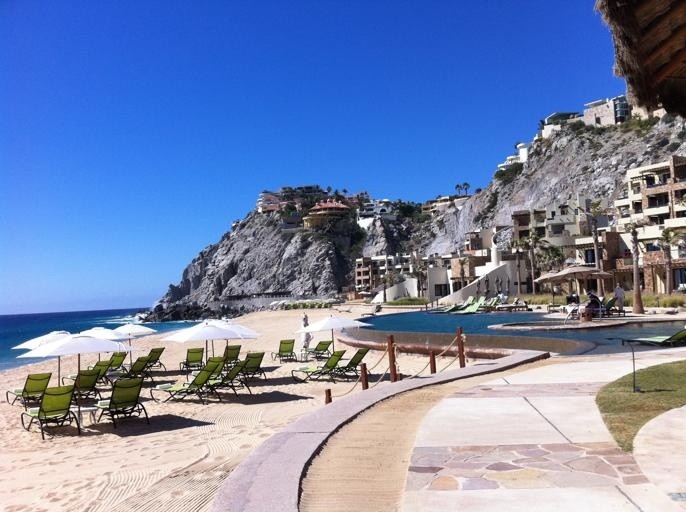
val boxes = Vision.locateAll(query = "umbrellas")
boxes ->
[476,273,510,302]
[159,317,258,364]
[295,315,375,353]
[302,312,313,350]
[10,323,158,426]
[532,265,614,306]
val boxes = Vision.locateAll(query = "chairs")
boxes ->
[547,297,625,319]
[6,371,52,411]
[163,344,268,403]
[124,355,152,380]
[85,358,117,388]
[145,346,168,373]
[18,385,81,440]
[429,296,527,314]
[316,347,370,380]
[299,339,332,361]
[62,370,101,405]
[108,351,131,376]
[271,339,298,365]
[291,349,346,385]
[91,376,150,428]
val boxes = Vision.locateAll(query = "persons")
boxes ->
[614,282,624,311]
